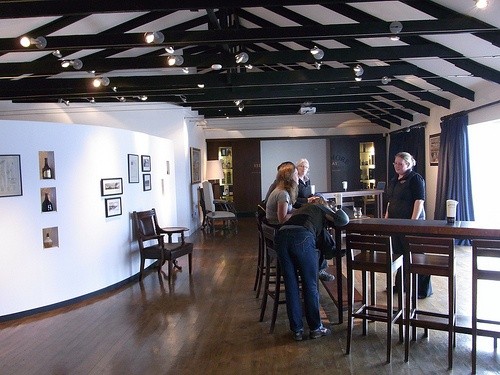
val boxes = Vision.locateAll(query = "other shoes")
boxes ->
[413,291,432,299]
[387,286,402,293]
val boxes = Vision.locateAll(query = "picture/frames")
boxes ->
[191,147,201,184]
[105,197,122,217]
[141,156,150,171]
[128,154,139,183]
[0,155,22,197]
[428,133,441,165]
[102,178,123,195]
[143,174,151,191]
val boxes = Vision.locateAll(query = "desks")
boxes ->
[321,217,500,347]
[155,227,189,270]
[318,189,384,216]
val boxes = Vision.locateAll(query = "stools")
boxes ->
[254,202,301,332]
[346,234,500,375]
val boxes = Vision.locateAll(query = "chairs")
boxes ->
[133,208,193,288]
[203,181,236,235]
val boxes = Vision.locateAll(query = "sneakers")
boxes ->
[295,327,304,341]
[324,247,347,260]
[310,323,332,339]
[318,269,336,282]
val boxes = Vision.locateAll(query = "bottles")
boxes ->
[220,147,233,200]
[42,158,51,178]
[43,193,53,211]
[44,233,52,248]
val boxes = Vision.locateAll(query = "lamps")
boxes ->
[20,32,390,110]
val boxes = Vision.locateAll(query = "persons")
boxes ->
[277,198,334,341]
[265,161,293,203]
[267,163,335,282]
[292,158,311,208]
[385,151,434,298]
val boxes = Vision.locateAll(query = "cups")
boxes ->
[354,206,362,218]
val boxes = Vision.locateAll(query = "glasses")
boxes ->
[393,161,404,166]
